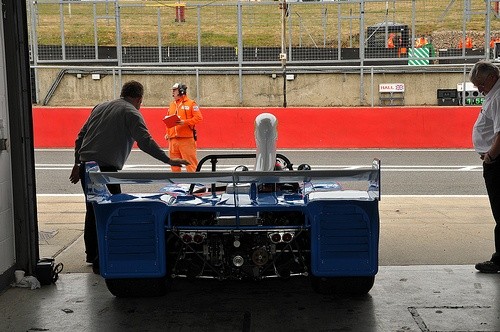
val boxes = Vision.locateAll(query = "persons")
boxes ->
[163,82,203,173]
[68,80,192,275]
[385,33,500,55]
[469,62,500,274]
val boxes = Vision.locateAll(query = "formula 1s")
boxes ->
[85,112,382,297]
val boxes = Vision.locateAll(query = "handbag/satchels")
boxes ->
[193,128,197,141]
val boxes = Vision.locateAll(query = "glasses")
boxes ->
[474,72,491,88]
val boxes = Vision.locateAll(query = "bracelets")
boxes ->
[487,152,494,161]
[75,163,81,167]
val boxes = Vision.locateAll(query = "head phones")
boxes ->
[178,82,184,96]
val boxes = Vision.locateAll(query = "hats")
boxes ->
[169,83,187,90]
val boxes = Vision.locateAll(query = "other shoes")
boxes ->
[474,261,500,273]
